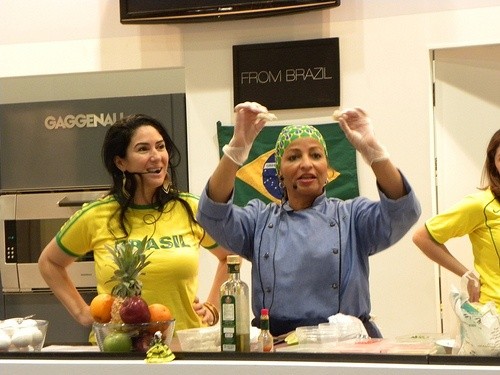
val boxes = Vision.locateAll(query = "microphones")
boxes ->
[129,169,160,175]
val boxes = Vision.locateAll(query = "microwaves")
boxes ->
[0,190,117,293]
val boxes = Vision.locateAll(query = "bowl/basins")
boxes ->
[177,326,260,352]
[93,320,175,353]
[296,325,339,350]
[0,320,49,353]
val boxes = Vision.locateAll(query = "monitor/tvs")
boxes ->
[118,0,340,24]
[231,36,341,112]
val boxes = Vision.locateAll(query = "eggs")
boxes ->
[0,318,44,351]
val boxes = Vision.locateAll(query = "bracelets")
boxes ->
[203,302,219,326]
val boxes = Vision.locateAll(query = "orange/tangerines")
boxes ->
[146,304,172,332]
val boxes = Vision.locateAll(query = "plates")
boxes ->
[435,338,455,348]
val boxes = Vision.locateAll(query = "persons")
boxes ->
[39,113,242,345]
[412,129,500,320]
[195,101,421,339]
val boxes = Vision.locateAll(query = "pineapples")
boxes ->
[104,235,151,335]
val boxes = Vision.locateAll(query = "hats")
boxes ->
[275,124,328,173]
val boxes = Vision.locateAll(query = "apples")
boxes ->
[133,332,156,351]
[90,294,116,323]
[120,295,151,325]
[103,331,133,352]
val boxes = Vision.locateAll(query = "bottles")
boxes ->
[220,255,250,353]
[258,309,273,352]
